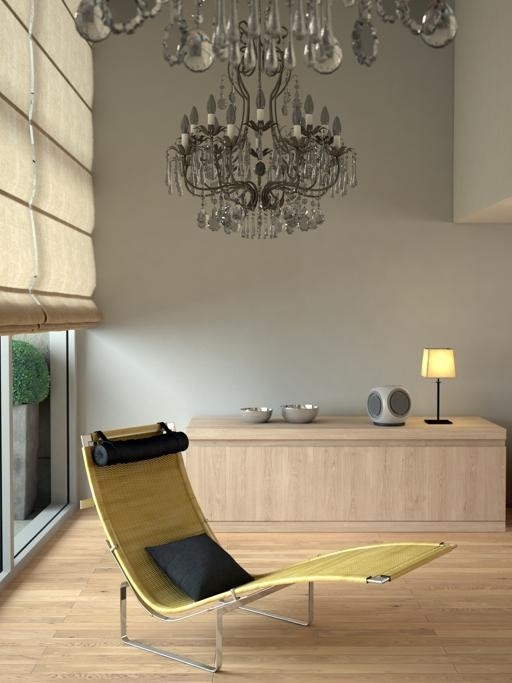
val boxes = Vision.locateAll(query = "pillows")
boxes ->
[144,532,255,602]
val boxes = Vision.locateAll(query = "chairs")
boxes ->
[80,422,461,672]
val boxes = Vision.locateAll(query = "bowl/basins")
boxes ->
[239,407,273,423]
[280,403,319,424]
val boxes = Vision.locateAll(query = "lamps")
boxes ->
[419,346,456,425]
[75,1,458,242]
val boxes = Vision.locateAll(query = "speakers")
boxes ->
[366,386,412,426]
